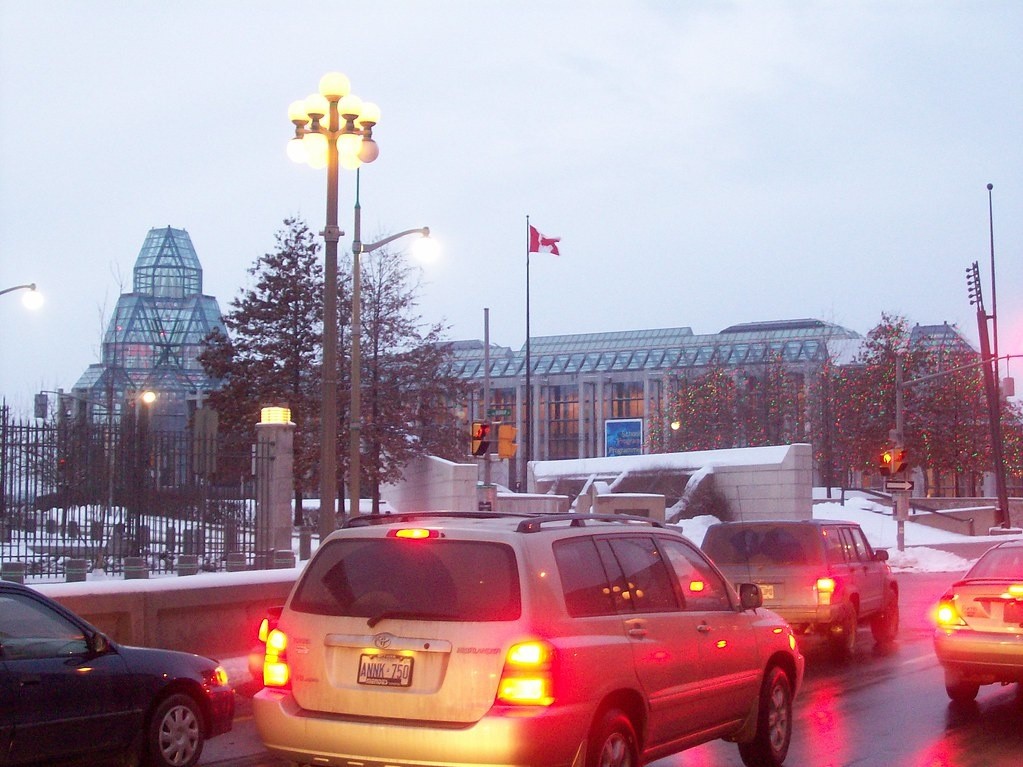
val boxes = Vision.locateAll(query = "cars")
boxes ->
[249,607,282,686]
[0,582,237,767]
[932,539,1023,700]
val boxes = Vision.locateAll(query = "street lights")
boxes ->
[349,226,439,524]
[289,70,379,547]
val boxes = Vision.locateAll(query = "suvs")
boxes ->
[700,521,899,657]
[253,511,803,767]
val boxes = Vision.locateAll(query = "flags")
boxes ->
[530,225,561,256]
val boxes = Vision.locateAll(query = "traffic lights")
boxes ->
[35,393,49,418]
[878,450,893,478]
[497,425,518,459]
[892,449,907,474]
[471,422,490,456]
